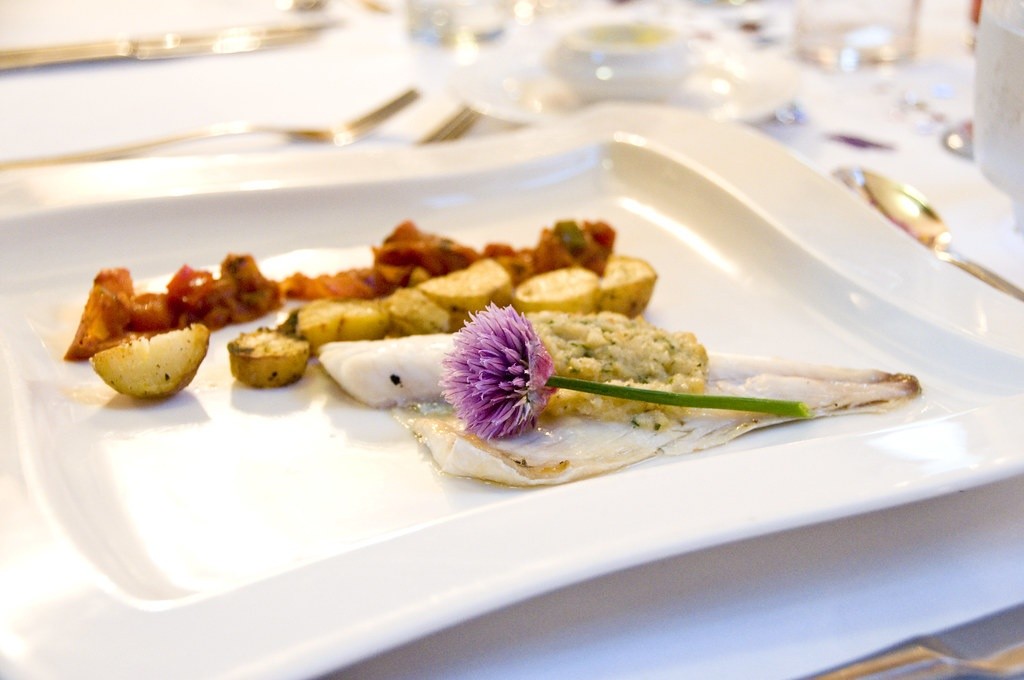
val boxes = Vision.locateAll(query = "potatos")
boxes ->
[87,322,210,399]
[225,253,658,388]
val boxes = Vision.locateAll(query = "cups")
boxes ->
[794,0,923,66]
[973,1,1024,196]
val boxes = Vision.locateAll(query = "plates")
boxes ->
[0,102,1024,680]
[457,55,797,124]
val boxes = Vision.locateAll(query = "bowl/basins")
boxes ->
[560,21,684,100]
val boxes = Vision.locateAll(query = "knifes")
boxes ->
[0,26,322,71]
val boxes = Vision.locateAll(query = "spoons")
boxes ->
[832,167,1024,303]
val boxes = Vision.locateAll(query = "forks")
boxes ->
[0,89,422,173]
[418,106,480,143]
